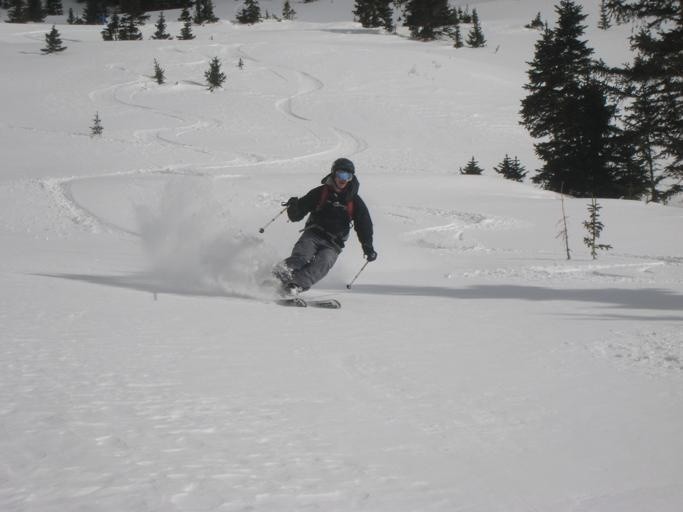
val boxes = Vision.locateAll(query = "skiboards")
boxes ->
[267,270,339,309]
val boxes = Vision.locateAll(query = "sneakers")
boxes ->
[284,283,303,296]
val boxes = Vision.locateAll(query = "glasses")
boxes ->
[335,172,353,182]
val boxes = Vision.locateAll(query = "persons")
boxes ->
[271,157,377,299]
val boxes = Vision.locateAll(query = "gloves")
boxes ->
[287,197,299,214]
[363,247,377,261]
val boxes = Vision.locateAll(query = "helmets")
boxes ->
[331,158,355,180]
